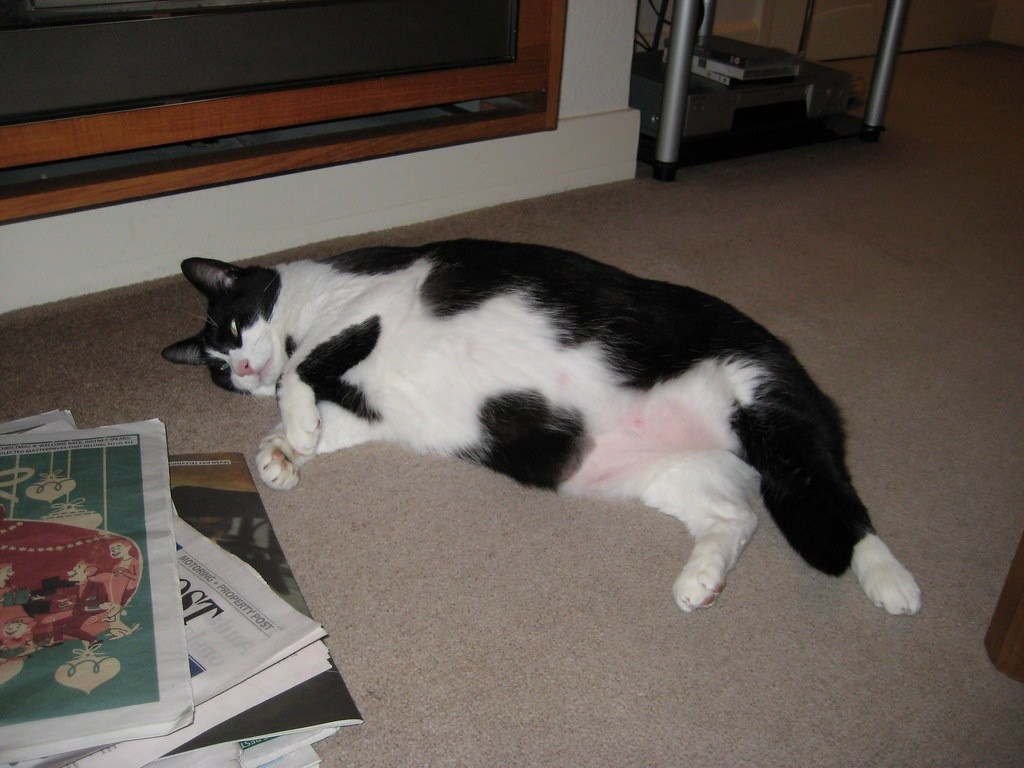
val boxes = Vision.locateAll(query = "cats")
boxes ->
[162,239,923,617]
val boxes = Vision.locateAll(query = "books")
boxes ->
[155,453,363,759]
